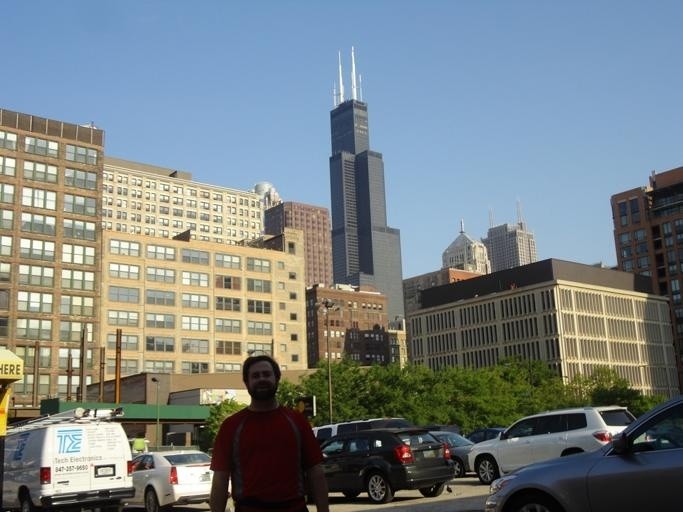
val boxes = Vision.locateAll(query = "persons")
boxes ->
[210,356,329,512]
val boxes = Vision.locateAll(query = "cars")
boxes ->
[123,449,234,511]
[429,428,480,475]
[468,424,506,449]
[483,395,683,511]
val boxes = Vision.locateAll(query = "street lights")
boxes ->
[149,373,161,451]
[324,292,342,423]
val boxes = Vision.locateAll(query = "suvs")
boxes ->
[307,429,457,507]
[468,404,646,484]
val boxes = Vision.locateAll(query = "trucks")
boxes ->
[1,405,137,512]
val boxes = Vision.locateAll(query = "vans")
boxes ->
[309,417,414,450]
